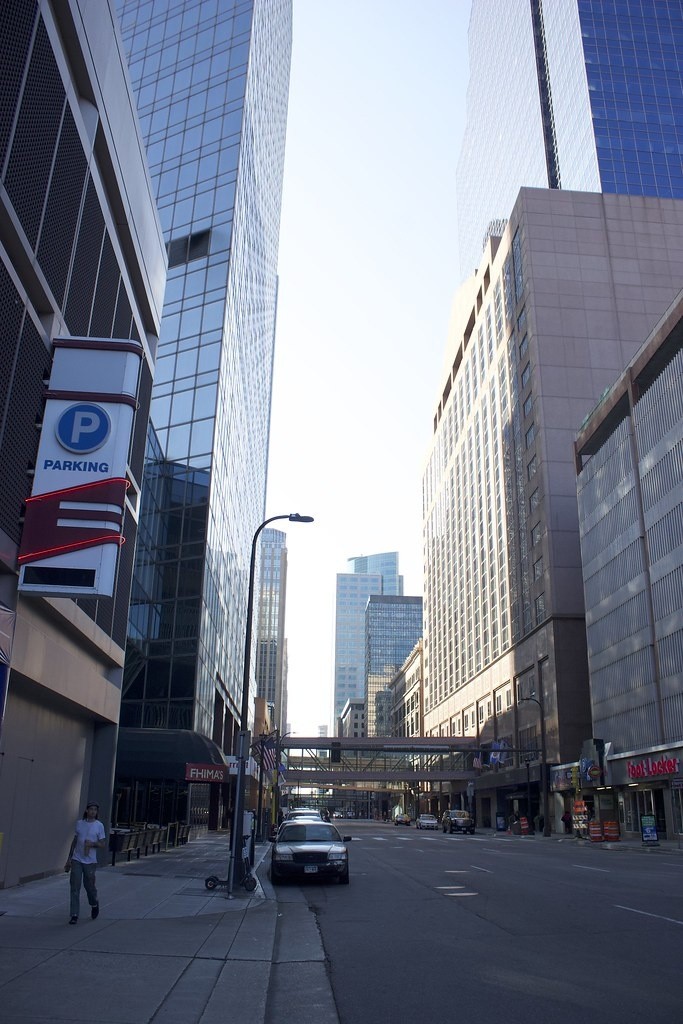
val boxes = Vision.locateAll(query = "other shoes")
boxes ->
[69,917,77,923]
[91,902,99,918]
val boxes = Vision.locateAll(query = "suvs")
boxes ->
[441,811,476,835]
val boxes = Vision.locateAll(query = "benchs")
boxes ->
[172,825,191,847]
[117,822,147,831]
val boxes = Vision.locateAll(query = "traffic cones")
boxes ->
[506,827,512,835]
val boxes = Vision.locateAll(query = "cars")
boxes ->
[394,815,411,826]
[276,807,325,836]
[346,813,355,818]
[333,812,342,819]
[268,822,352,884]
[415,814,439,830]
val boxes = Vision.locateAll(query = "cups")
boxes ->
[85,840,90,856]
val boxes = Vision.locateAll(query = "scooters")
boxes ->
[205,834,257,892]
[322,814,331,823]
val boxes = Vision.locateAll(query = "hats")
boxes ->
[87,801,99,808]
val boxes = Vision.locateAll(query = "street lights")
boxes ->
[228,513,316,899]
[275,732,297,834]
[257,729,279,839]
[520,697,550,837]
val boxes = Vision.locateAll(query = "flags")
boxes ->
[490,742,501,763]
[473,750,483,769]
[499,739,510,763]
[256,737,288,775]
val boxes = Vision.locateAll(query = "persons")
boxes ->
[514,811,520,821]
[64,802,106,924]
